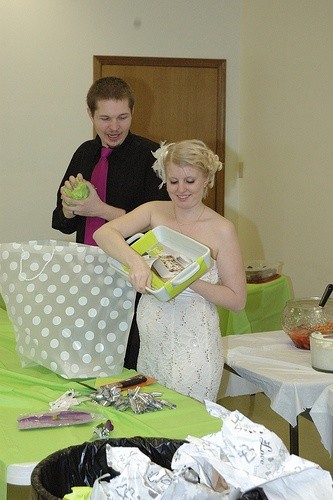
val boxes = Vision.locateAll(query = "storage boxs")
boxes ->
[106,224,211,302]
[245,261,280,281]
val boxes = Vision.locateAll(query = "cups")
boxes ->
[309,330,333,373]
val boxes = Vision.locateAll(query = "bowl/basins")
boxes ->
[244,259,284,283]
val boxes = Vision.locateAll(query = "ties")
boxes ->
[84,148,114,245]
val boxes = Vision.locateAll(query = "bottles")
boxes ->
[280,296,333,351]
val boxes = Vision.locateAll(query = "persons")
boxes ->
[91,138,247,406]
[51,76,175,373]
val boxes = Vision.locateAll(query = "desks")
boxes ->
[0,309,333,500]
[216,276,295,335]
[213,328,333,456]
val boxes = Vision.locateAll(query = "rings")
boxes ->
[73,210,75,215]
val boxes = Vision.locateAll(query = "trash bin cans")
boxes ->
[30,436,268,500]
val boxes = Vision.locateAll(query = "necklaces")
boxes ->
[173,203,206,235]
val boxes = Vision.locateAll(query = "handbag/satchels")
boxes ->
[1,239,136,381]
